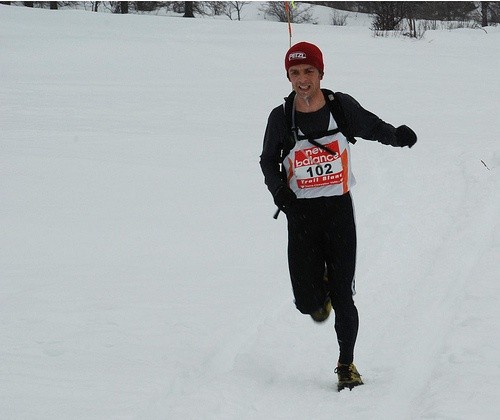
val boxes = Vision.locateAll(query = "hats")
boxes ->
[285,42,324,74]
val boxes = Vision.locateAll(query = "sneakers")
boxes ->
[335,360,363,392]
[310,271,332,322]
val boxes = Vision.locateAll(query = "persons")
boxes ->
[260,42,418,391]
